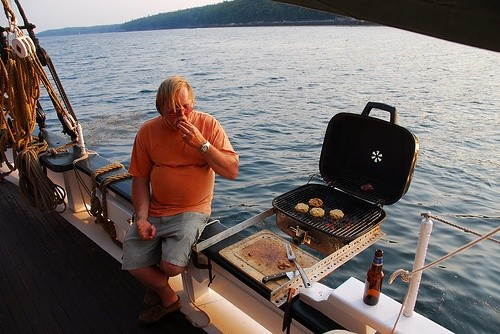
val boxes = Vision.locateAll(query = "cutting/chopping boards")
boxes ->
[218,229,321,292]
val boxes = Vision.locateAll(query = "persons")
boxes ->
[120,75,239,325]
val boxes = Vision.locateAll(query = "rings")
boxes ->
[182,134,186,138]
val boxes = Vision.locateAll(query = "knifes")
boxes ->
[262,267,310,284]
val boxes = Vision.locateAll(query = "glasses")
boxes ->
[165,107,194,116]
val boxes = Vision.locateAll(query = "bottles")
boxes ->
[363,248,385,306]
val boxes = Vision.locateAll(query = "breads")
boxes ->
[295,198,344,219]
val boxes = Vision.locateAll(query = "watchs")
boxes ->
[200,140,211,153]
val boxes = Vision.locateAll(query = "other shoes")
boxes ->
[139,295,181,323]
[143,277,169,305]
